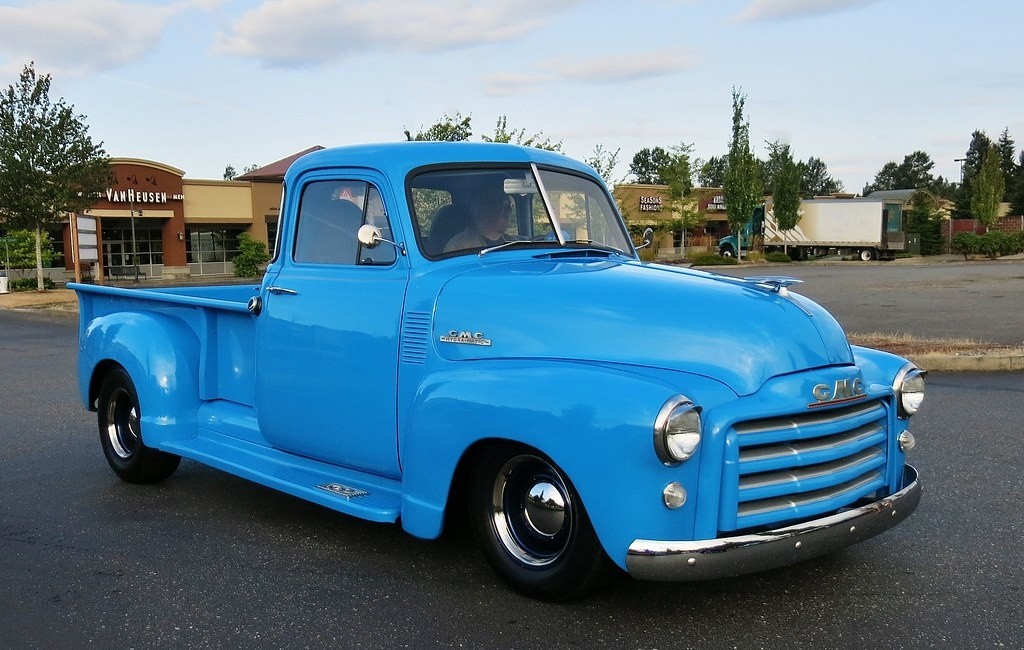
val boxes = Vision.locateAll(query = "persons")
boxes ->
[442,186,529,259]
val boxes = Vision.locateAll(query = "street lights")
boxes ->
[954,159,966,185]
[130,207,143,281]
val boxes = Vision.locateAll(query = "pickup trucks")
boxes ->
[65,142,932,601]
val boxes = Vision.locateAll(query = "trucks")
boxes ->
[720,199,906,263]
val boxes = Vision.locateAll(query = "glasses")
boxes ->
[487,199,512,208]
